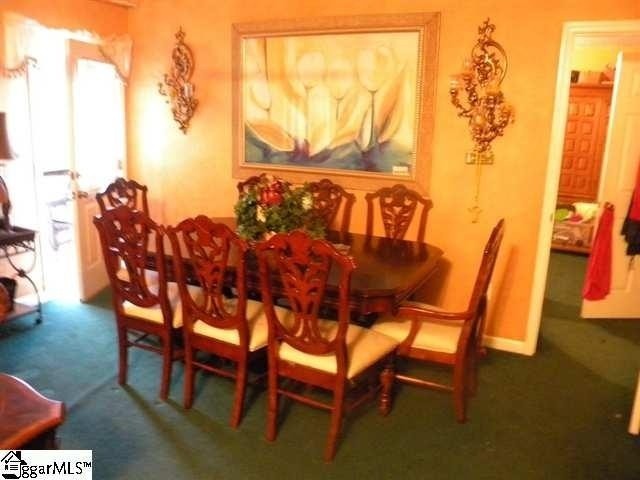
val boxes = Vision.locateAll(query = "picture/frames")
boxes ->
[231,12,442,199]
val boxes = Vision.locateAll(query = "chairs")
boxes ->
[92,204,227,398]
[365,182,434,242]
[95,177,161,289]
[296,177,356,231]
[253,230,399,465]
[369,219,505,423]
[237,173,291,197]
[165,215,296,430]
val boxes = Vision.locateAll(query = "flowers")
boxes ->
[233,175,328,241]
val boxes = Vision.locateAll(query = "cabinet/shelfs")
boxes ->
[0,225,46,327]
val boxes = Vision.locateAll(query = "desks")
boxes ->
[0,370,66,449]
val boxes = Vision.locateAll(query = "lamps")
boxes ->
[155,25,198,136]
[449,18,515,164]
[0,112,13,233]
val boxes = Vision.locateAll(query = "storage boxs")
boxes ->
[580,71,600,84]
